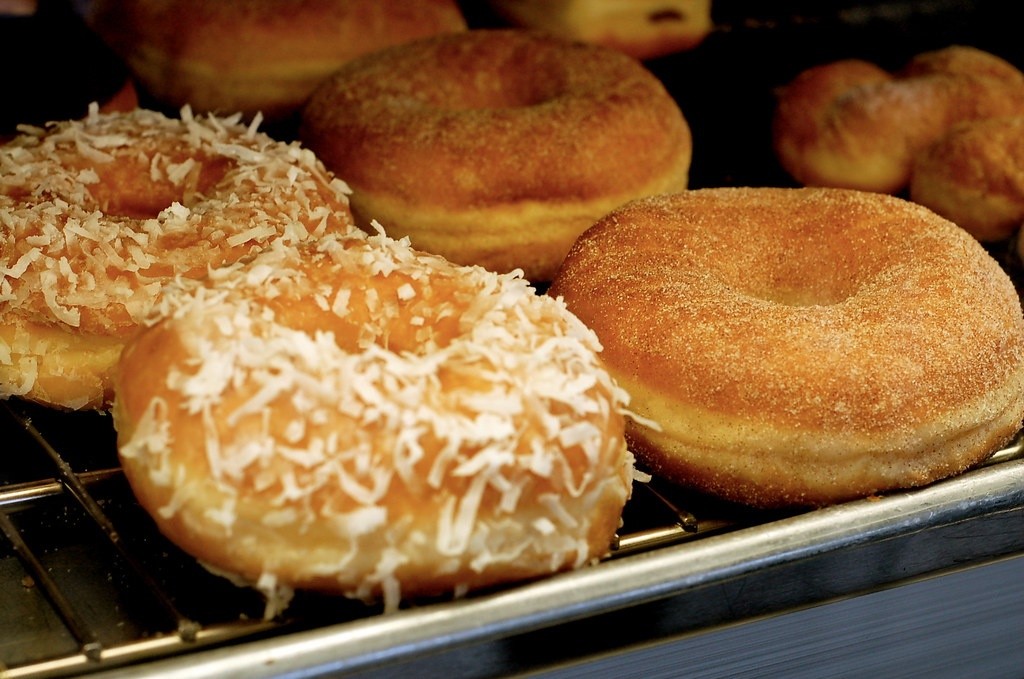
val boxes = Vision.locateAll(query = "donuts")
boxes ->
[0,0,1024,612]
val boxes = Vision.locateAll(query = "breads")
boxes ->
[497,0,712,56]
[775,46,1024,265]
